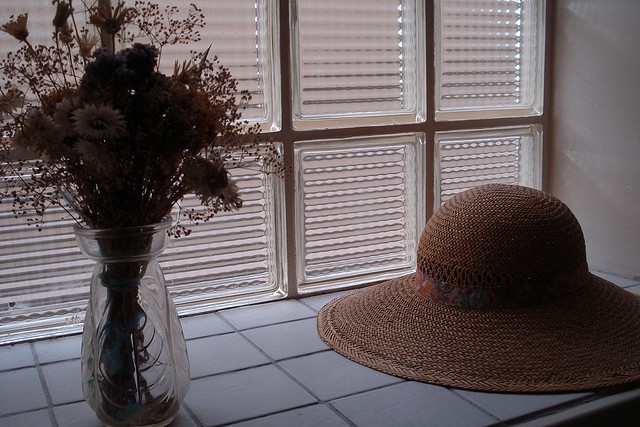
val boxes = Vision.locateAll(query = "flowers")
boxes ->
[0,0,294,416]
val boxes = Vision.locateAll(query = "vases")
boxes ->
[72,215,191,427]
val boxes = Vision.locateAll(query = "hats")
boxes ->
[316,183,640,393]
